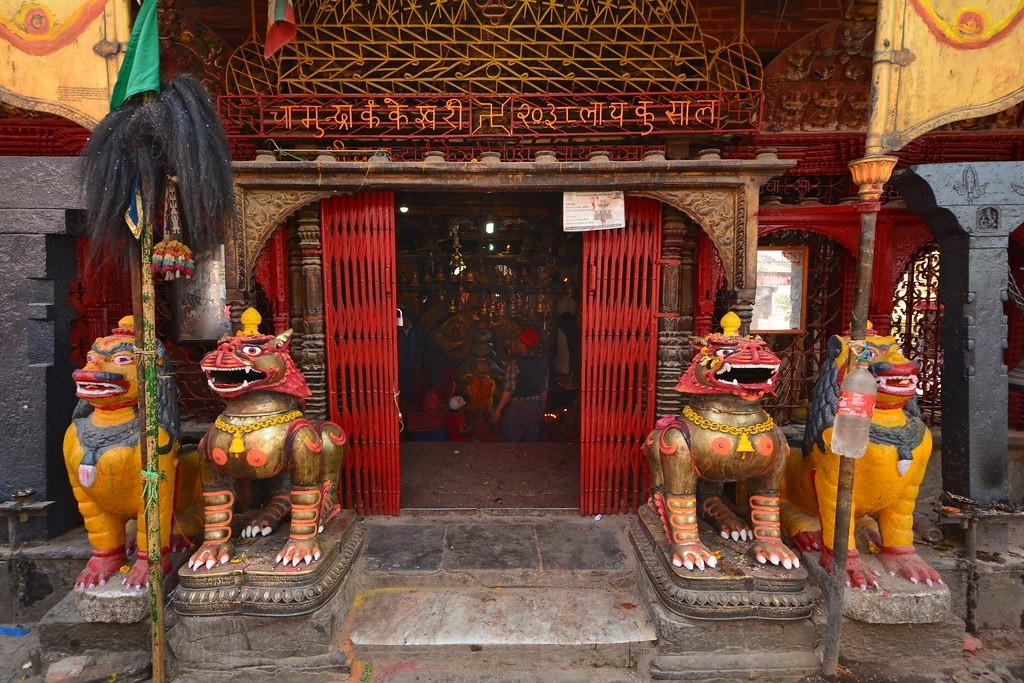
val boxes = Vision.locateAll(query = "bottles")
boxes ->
[830,358,877,460]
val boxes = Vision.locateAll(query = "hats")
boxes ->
[520,328,540,348]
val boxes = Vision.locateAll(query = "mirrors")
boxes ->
[172,236,233,347]
[749,246,809,334]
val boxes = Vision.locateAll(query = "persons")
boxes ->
[405,344,472,443]
[452,313,517,381]
[491,328,547,443]
[397,307,424,406]
